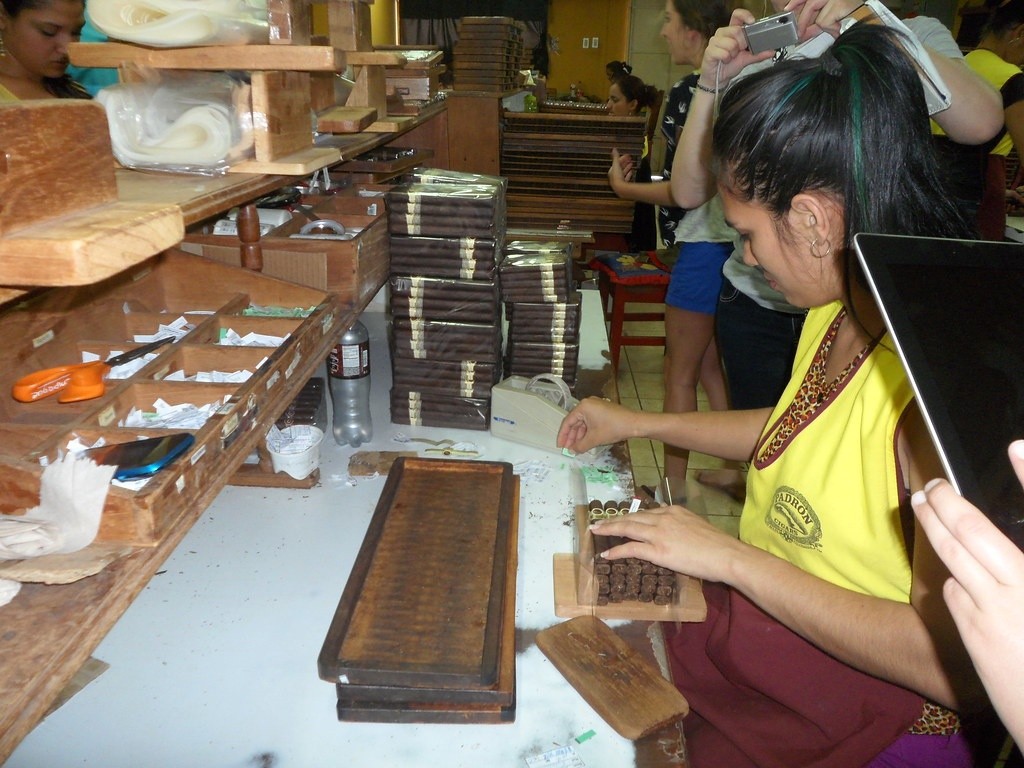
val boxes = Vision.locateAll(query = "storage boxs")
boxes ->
[175,181,395,313]
[0,248,341,547]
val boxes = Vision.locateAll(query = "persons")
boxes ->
[606,60,658,253]
[927,0,1024,214]
[608,0,747,506]
[0,0,95,100]
[670,0,1005,408]
[557,14,995,767]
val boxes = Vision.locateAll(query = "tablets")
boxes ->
[853,232,1024,554]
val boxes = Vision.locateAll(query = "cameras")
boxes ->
[743,10,798,55]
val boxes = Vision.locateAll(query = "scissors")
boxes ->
[10,335,175,403]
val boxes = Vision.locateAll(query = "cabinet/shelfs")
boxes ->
[0,100,449,768]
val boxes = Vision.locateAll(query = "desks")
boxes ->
[0,289,696,768]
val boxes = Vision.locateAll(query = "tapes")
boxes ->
[524,373,572,410]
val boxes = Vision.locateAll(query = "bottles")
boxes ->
[523,93,537,114]
[326,320,373,449]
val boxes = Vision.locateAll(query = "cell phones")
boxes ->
[81,432,196,481]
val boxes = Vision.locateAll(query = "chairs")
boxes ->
[588,250,672,379]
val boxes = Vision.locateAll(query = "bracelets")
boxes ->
[696,80,728,94]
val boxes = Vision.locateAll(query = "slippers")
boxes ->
[692,470,746,493]
[633,485,688,505]
